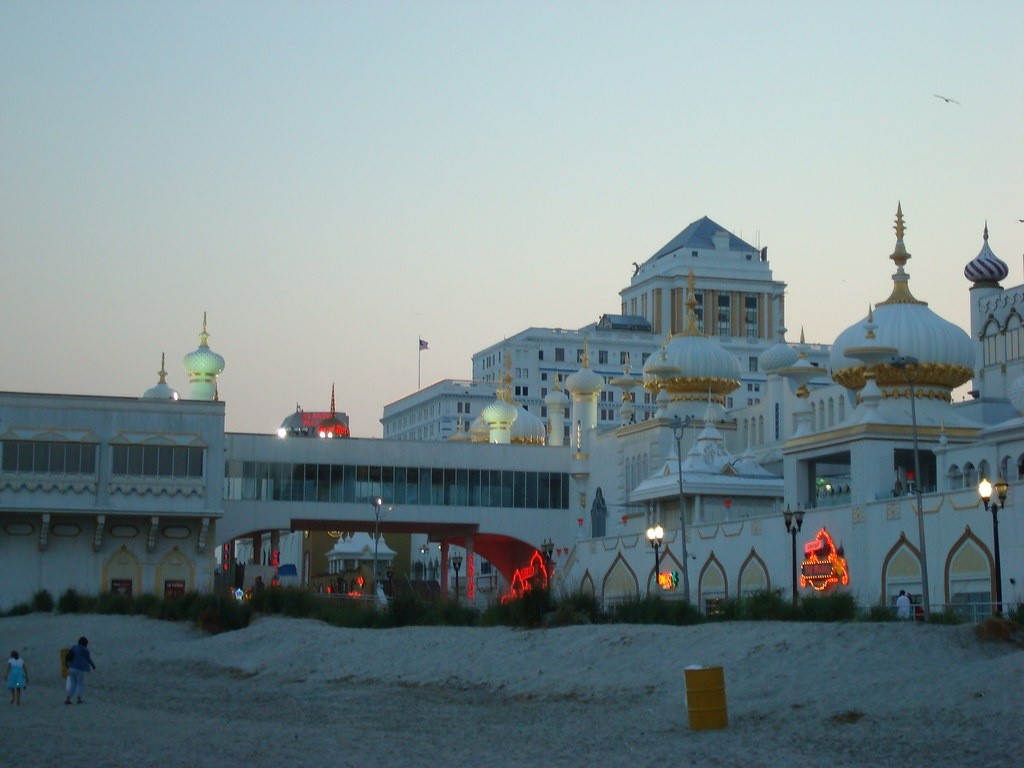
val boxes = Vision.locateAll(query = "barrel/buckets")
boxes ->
[59,645,77,679]
[683,665,729,731]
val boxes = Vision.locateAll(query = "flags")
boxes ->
[419,340,428,350]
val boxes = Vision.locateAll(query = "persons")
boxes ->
[64,636,96,704]
[896,590,911,619]
[251,576,265,595]
[4,650,30,706]
